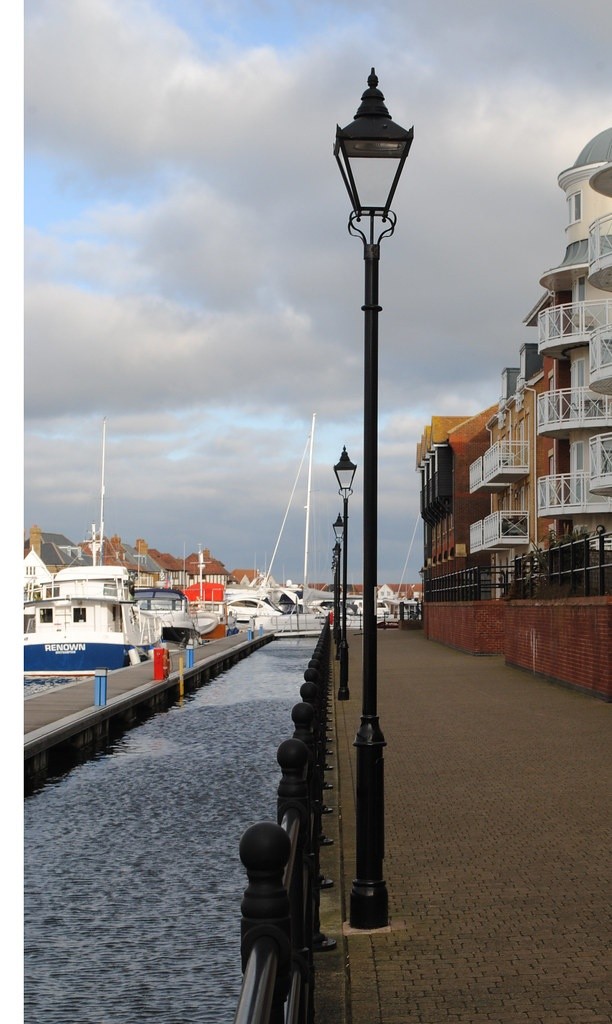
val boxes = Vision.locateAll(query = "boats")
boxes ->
[23,414,161,677]
[128,542,423,649]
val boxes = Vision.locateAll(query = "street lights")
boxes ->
[331,513,343,660]
[330,62,416,931]
[332,445,359,700]
